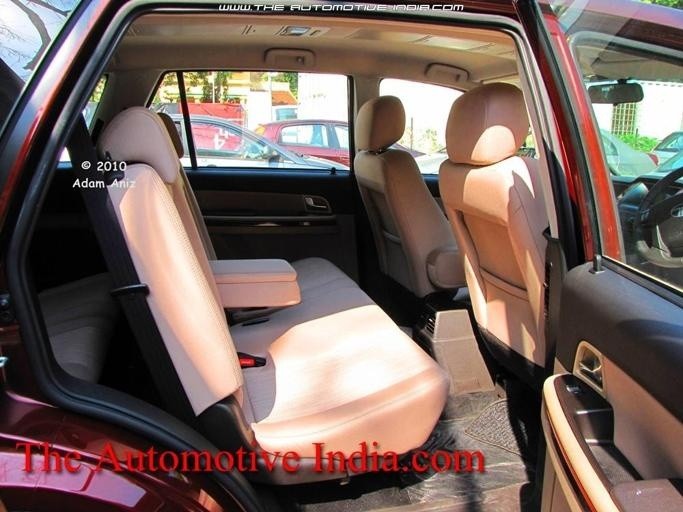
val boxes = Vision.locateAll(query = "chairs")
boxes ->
[148,108,378,313]
[99,111,449,490]
[353,95,498,298]
[436,75,618,389]
